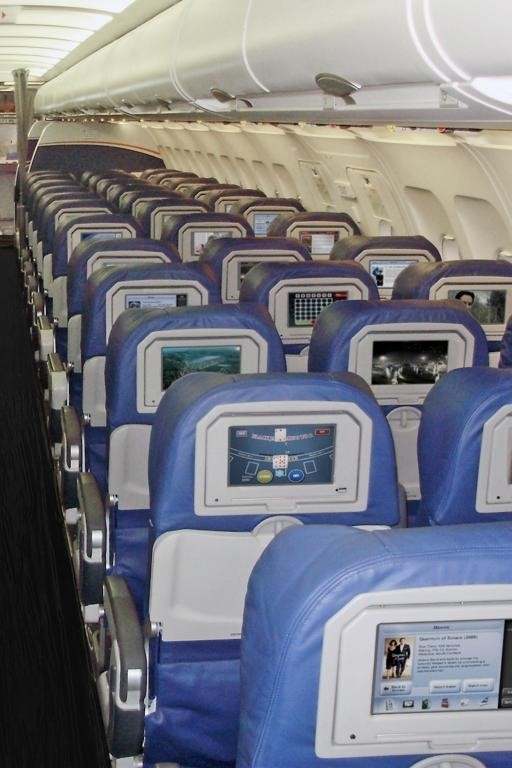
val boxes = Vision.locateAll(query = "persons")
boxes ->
[383,356,445,386]
[385,640,397,680]
[396,637,410,678]
[455,291,474,312]
[376,270,383,286]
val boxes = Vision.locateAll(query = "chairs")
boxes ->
[236,524,512,762]
[239,260,382,373]
[500,314,512,371]
[265,210,363,262]
[60,265,232,526]
[415,366,510,523]
[80,300,288,605]
[109,367,400,765]
[391,259,511,367]
[17,164,307,458]
[307,297,493,519]
[327,234,443,302]
